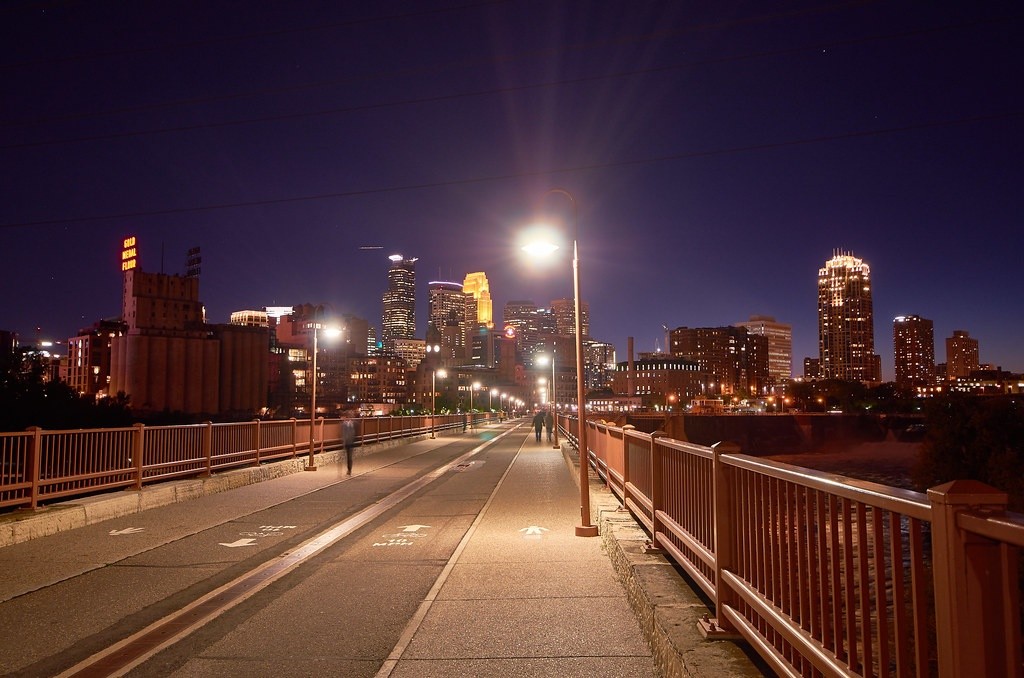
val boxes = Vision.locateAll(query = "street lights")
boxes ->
[509,395,515,419]
[817,398,826,416]
[538,377,553,433]
[539,386,549,409]
[533,347,560,449]
[507,188,599,537]
[305,302,343,471]
[429,360,447,439]
[500,392,506,423]
[769,396,777,415]
[782,398,789,413]
[489,387,498,425]
[470,377,481,429]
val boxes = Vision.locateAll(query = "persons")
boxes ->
[498,409,504,423]
[531,410,554,443]
[462,411,468,434]
